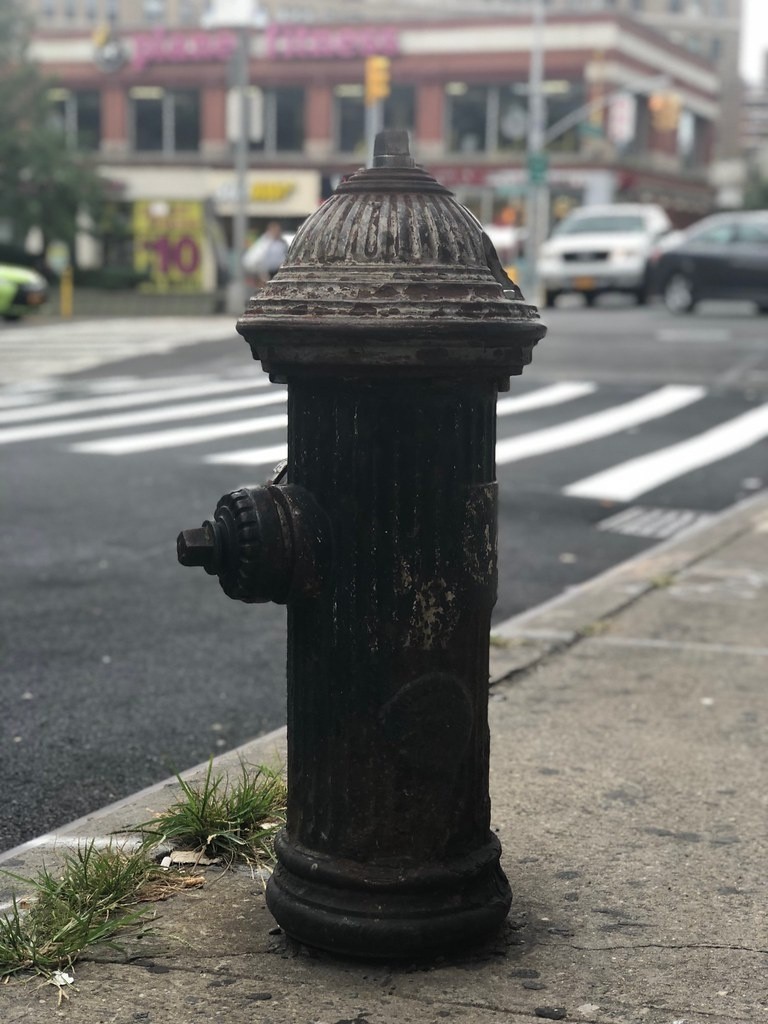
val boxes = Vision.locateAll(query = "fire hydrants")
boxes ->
[174,128,518,958]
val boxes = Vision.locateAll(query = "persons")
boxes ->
[240,218,291,290]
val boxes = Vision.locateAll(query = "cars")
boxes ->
[649,210,768,316]
[539,205,682,305]
[523,76,677,300]
[1,260,51,322]
